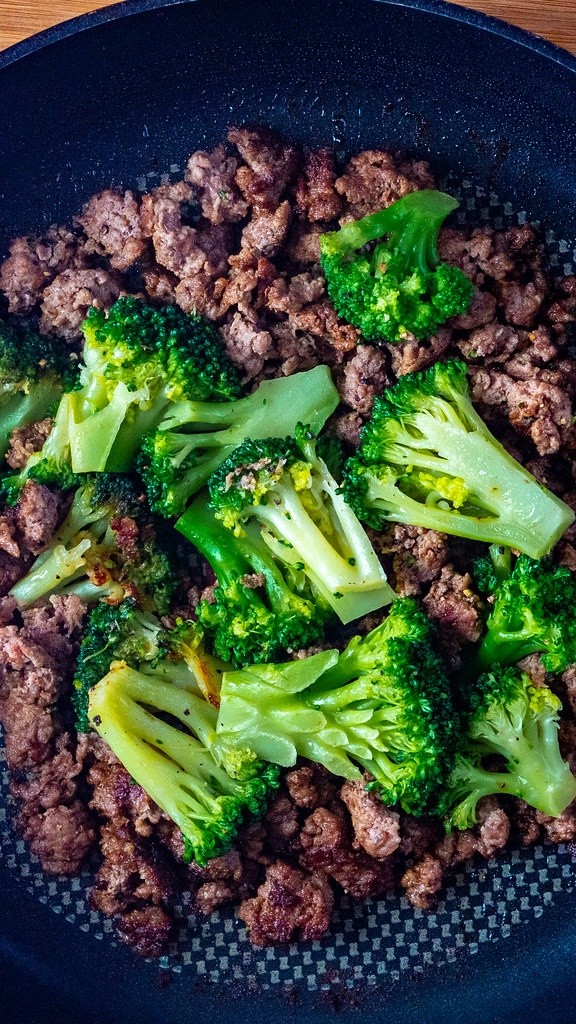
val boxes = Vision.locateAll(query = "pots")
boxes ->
[0,0,576,1024]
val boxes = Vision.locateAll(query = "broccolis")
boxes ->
[0,188,576,866]
